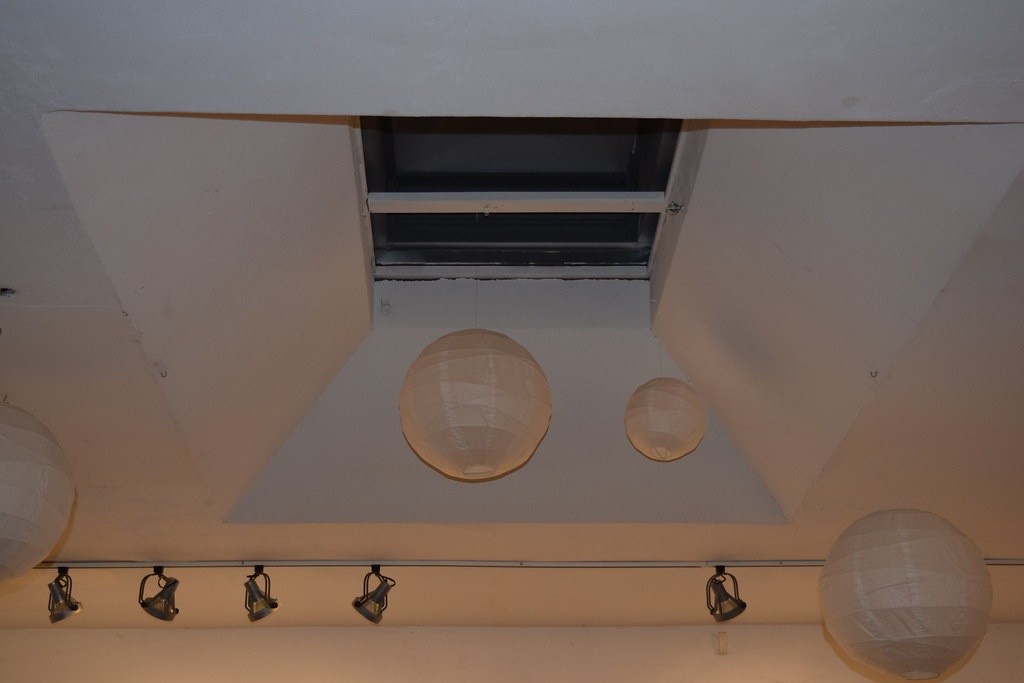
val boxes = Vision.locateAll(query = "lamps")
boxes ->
[48,568,81,623]
[243,565,278,622]
[352,565,396,624]
[706,565,747,622]
[400,266,553,480]
[139,567,179,621]
[625,378,705,461]
[818,371,992,679]
[0,289,76,579]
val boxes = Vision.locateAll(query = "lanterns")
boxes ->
[625,377,707,460]
[0,402,75,585]
[400,329,550,481]
[820,509,992,680]
